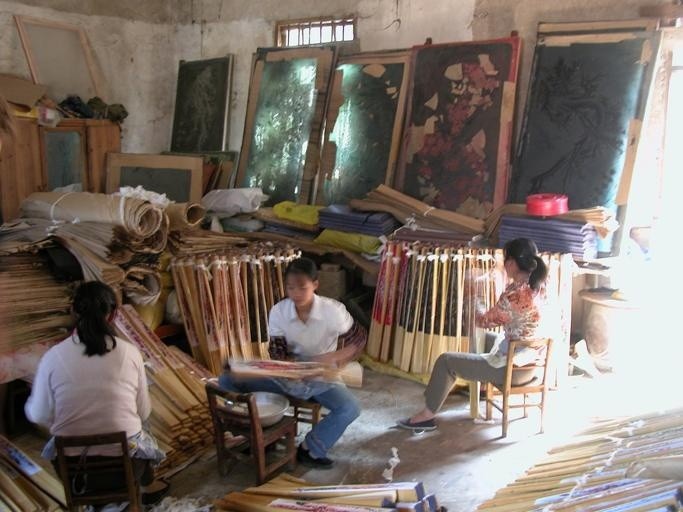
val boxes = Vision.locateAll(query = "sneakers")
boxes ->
[396,416,436,433]
[295,442,336,470]
[140,476,171,508]
[458,385,486,400]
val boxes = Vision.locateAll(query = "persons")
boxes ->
[215,256,367,468]
[22,280,167,510]
[397,237,550,430]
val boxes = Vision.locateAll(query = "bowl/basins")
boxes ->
[224,390,290,429]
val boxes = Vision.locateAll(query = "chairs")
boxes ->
[205,381,297,487]
[288,338,344,430]
[486,337,554,437]
[54,431,146,511]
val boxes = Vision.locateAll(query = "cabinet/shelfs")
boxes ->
[40,118,121,194]
